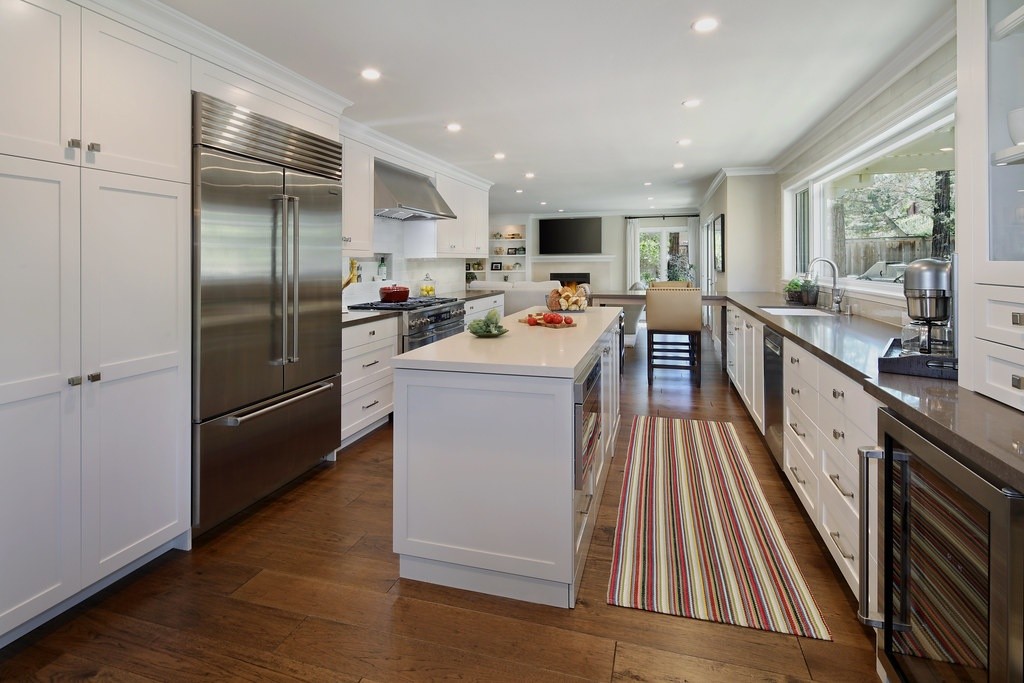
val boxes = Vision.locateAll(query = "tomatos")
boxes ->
[528,312,573,326]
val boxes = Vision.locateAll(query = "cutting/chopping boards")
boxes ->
[518,313,578,328]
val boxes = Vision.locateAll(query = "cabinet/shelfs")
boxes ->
[390,305,624,609]
[323,135,531,461]
[955,1,1024,415]
[726,301,766,438]
[0,1,193,652]
[783,337,889,634]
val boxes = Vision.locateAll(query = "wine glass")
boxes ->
[478,266,482,271]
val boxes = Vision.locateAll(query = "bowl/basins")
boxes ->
[544,294,590,313]
[1007,107,1024,145]
[373,275,382,281]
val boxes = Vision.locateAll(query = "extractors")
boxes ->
[374,157,457,221]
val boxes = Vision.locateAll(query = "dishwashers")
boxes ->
[763,324,784,471]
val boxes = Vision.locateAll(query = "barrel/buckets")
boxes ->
[418,272,437,297]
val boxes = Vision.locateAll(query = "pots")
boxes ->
[379,282,410,303]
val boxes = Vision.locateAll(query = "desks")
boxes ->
[589,290,727,308]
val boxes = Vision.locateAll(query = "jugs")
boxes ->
[513,262,522,270]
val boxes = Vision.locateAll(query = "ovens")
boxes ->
[403,319,466,354]
[191,90,342,533]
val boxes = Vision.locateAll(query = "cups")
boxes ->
[473,264,478,271]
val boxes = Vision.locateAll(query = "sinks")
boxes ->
[758,306,838,316]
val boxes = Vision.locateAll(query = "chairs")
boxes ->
[645,281,702,388]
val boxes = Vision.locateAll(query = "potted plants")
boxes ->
[784,272,822,307]
[473,262,481,270]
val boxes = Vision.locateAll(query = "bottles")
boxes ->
[357,264,362,283]
[378,256,387,281]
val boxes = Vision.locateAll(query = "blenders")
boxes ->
[899,257,953,359]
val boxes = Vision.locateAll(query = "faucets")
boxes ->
[803,257,846,312]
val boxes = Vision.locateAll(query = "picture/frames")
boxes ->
[713,214,725,273]
[491,262,502,270]
[466,263,471,270]
[508,248,516,255]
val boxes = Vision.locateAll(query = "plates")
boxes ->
[469,328,509,337]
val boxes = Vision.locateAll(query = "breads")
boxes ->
[549,286,587,311]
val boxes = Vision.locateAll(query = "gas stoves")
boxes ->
[347,296,466,336]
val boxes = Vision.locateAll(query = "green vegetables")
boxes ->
[468,308,504,335]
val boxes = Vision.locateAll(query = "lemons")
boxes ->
[420,285,435,296]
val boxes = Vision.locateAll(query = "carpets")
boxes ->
[607,415,834,641]
